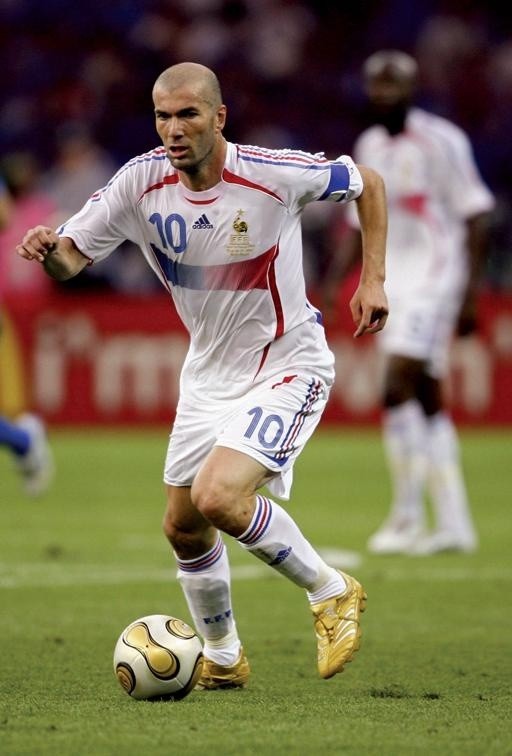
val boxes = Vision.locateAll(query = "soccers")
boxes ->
[114,614,203,701]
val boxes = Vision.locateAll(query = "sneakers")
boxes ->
[14,411,52,498]
[308,569,367,679]
[194,650,250,692]
[365,521,477,557]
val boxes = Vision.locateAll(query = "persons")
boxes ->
[0,411,56,500]
[300,45,498,560]
[2,2,505,336]
[17,57,393,698]
